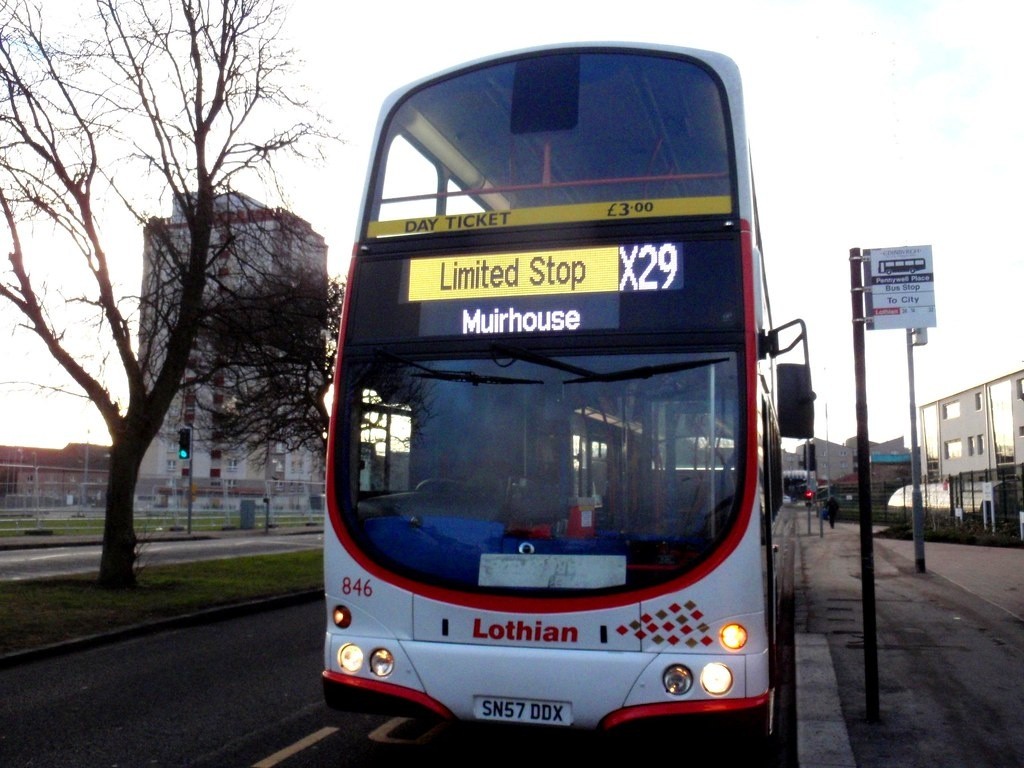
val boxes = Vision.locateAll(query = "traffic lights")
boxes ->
[179,427,192,460]
[804,489,813,499]
[803,444,815,470]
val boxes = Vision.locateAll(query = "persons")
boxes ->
[826,495,839,529]
[790,486,796,502]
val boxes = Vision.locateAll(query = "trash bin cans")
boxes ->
[240,500,255,529]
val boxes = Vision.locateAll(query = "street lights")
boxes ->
[908,327,926,572]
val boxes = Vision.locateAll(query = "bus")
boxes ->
[322,41,817,754]
